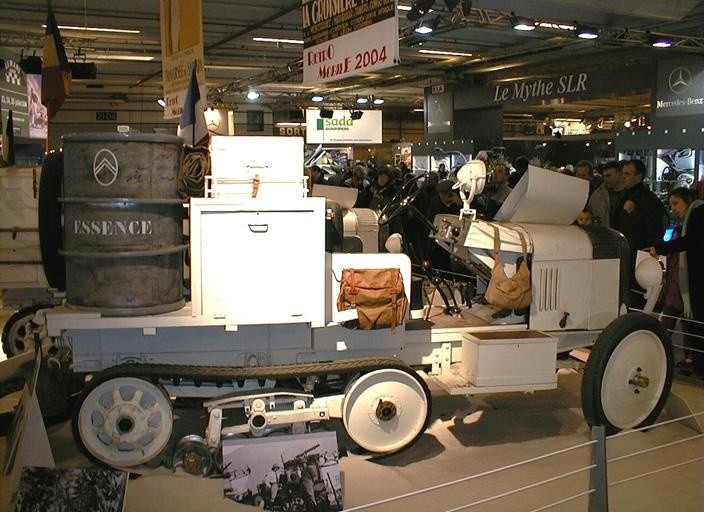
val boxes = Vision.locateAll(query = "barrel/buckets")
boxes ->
[57,131,187,317]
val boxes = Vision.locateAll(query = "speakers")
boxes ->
[23,56,43,74]
[69,61,96,79]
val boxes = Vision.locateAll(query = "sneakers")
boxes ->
[680,356,695,376]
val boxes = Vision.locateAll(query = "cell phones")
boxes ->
[663,227,675,241]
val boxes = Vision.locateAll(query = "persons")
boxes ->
[6,110,14,165]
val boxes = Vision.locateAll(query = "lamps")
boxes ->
[414,12,673,49]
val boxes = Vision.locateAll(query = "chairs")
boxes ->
[324,200,424,313]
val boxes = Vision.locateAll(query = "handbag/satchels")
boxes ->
[337,268,408,334]
[484,261,532,309]
